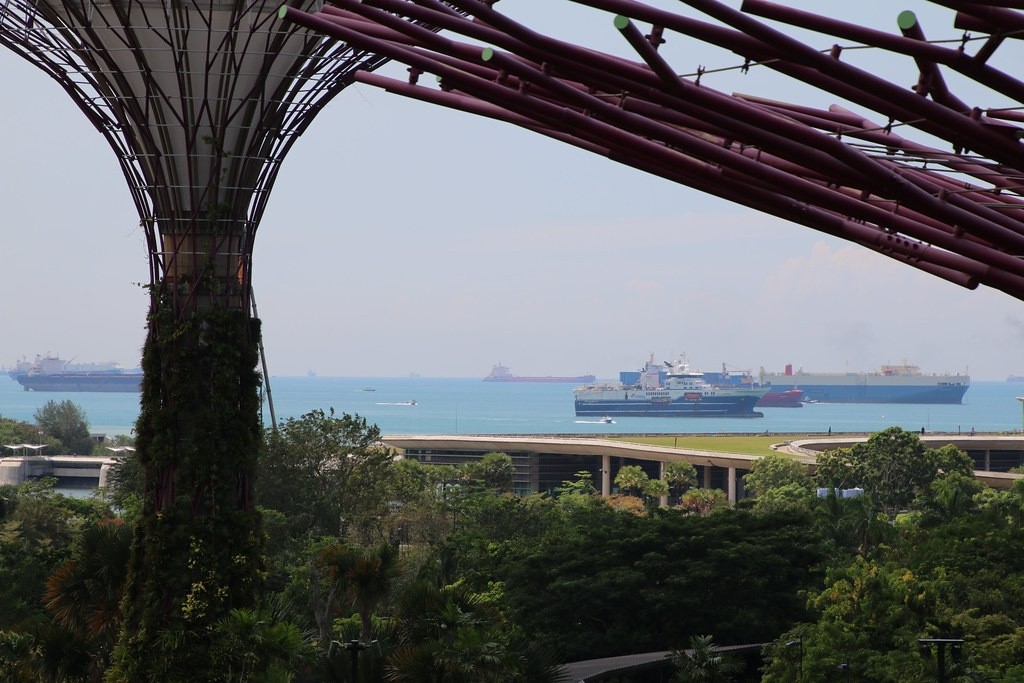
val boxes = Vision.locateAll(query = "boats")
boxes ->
[480,361,597,384]
[600,414,613,423]
[571,349,774,418]
[618,352,972,405]
[755,381,805,408]
[6,352,145,393]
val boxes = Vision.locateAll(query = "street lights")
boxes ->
[327,639,383,683]
[918,638,965,683]
[836,657,850,683]
[785,634,804,681]
